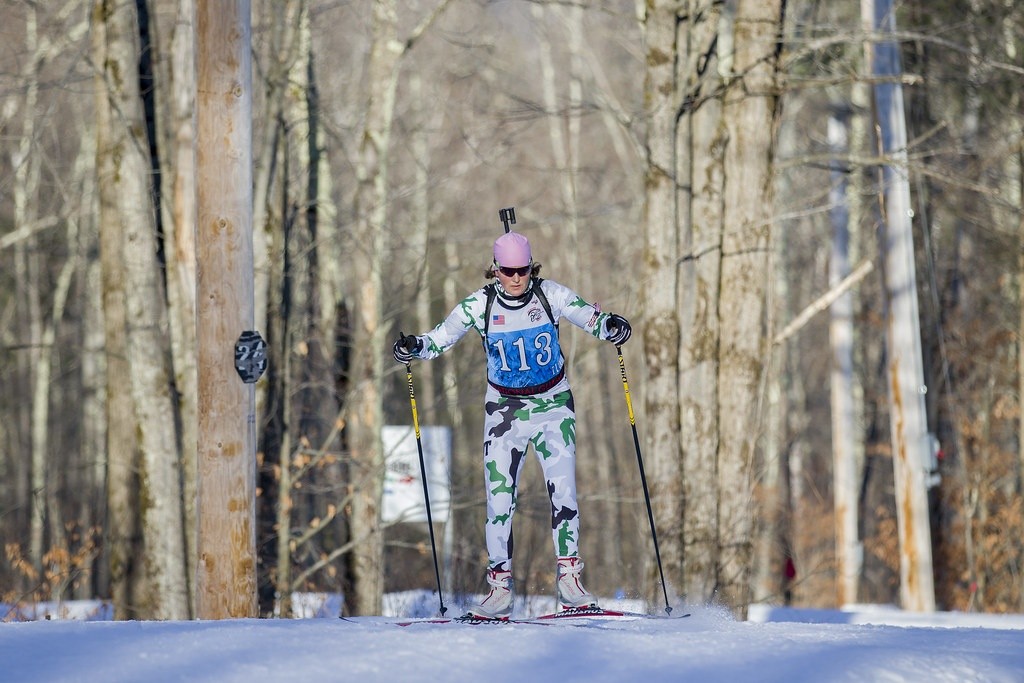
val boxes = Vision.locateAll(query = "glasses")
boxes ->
[499,266,531,277]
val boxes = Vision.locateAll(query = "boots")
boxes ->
[468,570,514,617]
[556,557,598,612]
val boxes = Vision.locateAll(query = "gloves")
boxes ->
[393,335,417,365]
[606,315,631,346]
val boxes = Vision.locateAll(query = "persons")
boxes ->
[394,232,631,618]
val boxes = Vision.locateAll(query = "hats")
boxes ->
[494,230,531,267]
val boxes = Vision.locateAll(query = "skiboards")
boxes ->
[336,605,694,627]
